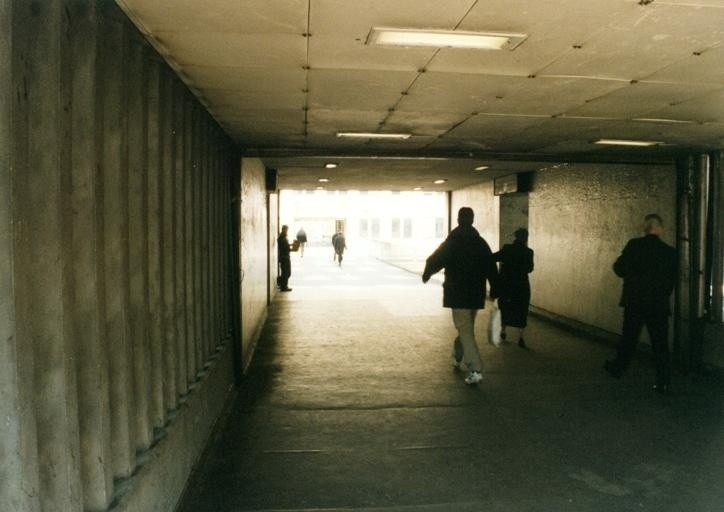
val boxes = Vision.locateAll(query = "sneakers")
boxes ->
[453,359,460,366]
[280,288,292,291]
[464,371,482,384]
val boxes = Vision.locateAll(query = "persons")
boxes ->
[333,229,346,263]
[603,212,678,393]
[422,206,498,386]
[276,223,308,292]
[492,229,536,350]
